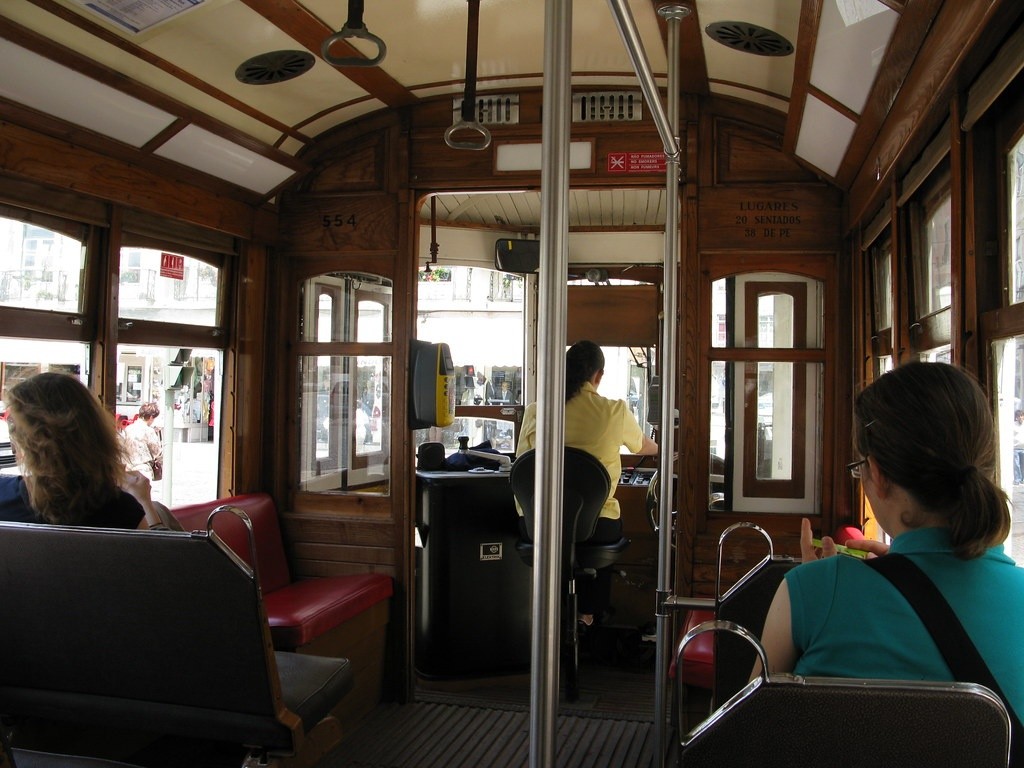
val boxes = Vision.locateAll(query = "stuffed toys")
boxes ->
[175,385,189,409]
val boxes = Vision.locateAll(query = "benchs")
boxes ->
[0,505,355,768]
[173,495,394,768]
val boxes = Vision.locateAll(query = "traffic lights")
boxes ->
[465,365,475,376]
[166,345,194,391]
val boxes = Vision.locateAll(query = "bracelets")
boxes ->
[149,522,163,528]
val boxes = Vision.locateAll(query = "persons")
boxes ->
[496,382,513,404]
[513,340,658,626]
[746,362,1024,732]
[1014,411,1024,485]
[0,372,185,532]
[119,402,163,482]
[361,380,374,407]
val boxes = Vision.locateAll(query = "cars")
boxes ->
[758,392,773,455]
[316,395,373,447]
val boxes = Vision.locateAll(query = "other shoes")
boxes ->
[577,606,615,640]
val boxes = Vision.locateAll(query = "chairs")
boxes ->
[671,520,1013,768]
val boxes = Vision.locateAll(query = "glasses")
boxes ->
[846,460,867,479]
